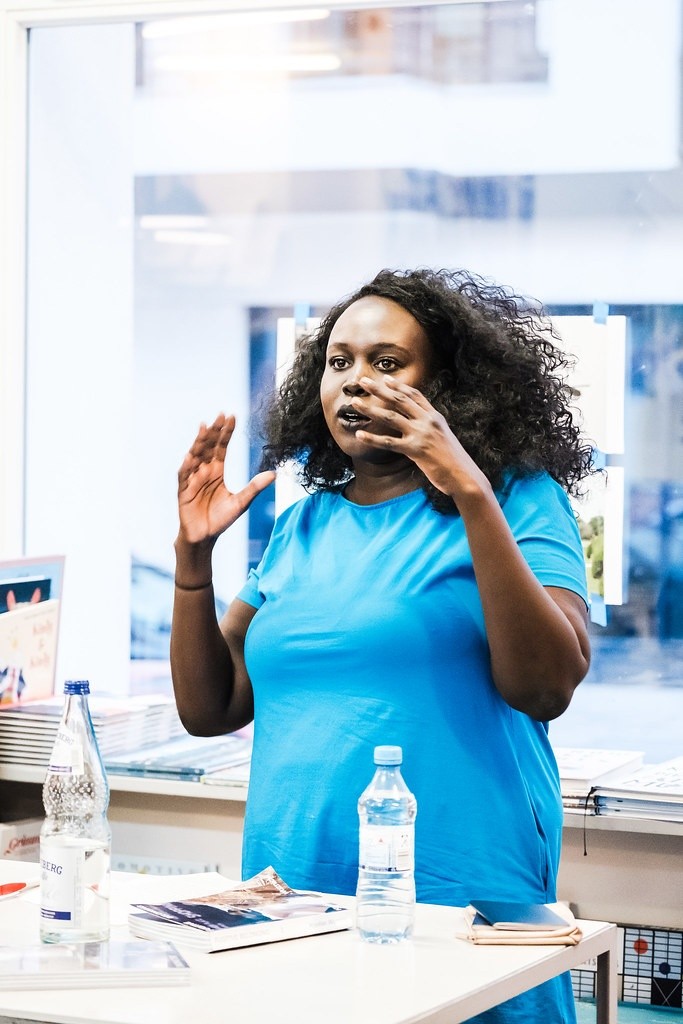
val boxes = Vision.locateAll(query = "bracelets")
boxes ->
[174,580,212,591]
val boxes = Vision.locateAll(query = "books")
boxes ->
[590,757,682,822]
[0,554,65,710]
[125,867,351,953]
[109,733,252,788]
[467,899,570,932]
[0,690,188,768]
[0,940,193,992]
[551,745,644,816]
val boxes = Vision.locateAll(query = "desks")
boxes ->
[1,764,683,1024]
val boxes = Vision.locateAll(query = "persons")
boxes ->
[171,271,605,1024]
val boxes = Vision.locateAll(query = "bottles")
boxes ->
[356,746,418,944]
[40,680,113,946]
